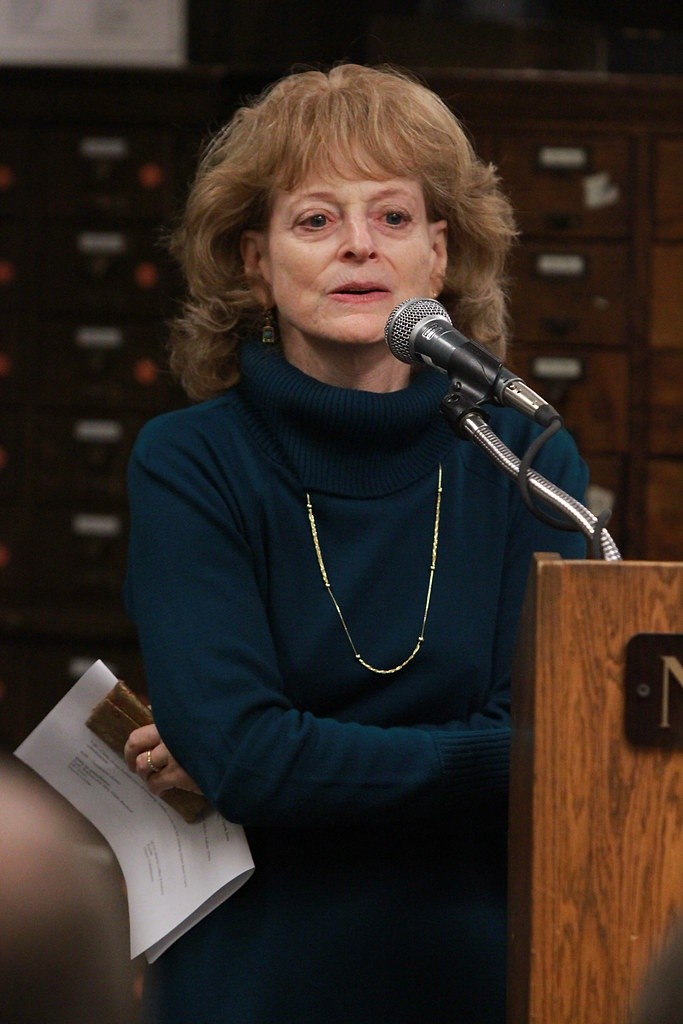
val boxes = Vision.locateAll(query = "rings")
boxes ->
[147,751,160,772]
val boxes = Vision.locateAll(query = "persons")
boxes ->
[125,63,589,1023]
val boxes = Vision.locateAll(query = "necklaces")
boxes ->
[307,464,443,674]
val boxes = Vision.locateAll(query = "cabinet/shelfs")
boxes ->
[0,58,683,737]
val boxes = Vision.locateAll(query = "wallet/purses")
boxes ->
[84,680,209,824]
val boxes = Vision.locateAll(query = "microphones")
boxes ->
[382,296,564,430]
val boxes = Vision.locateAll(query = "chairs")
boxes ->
[0,748,147,1024]
